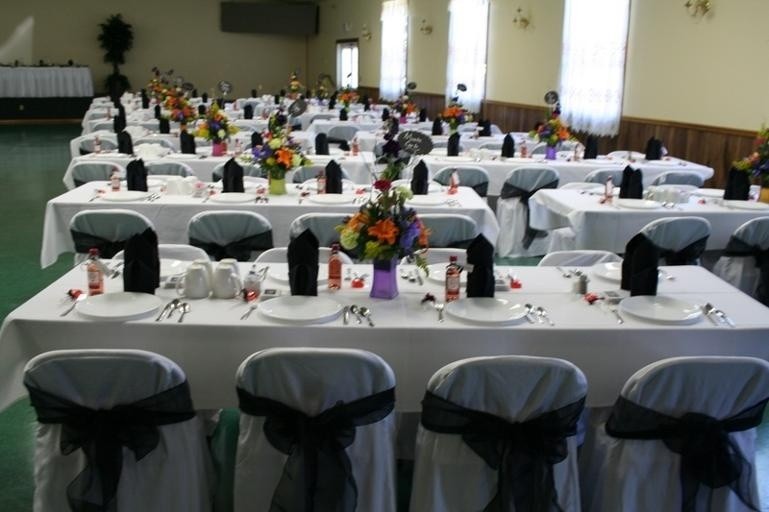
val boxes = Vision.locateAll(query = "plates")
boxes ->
[591,262,663,281]
[445,297,529,322]
[76,291,162,318]
[590,187,769,209]
[258,295,344,322]
[102,178,449,205]
[430,150,681,167]
[620,295,703,321]
[268,262,327,282]
[95,133,362,159]
[421,262,500,283]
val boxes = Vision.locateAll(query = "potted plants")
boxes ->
[96,13,135,96]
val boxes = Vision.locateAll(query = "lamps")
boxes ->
[685,1,712,16]
[512,7,529,28]
[419,19,433,35]
[362,24,371,41]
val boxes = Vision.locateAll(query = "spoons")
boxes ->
[705,303,724,326]
[715,310,736,327]
[350,305,363,324]
[167,299,183,321]
[524,303,540,322]
[178,303,192,322]
[537,307,554,327]
[359,306,374,328]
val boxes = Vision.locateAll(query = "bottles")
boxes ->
[88,249,103,296]
[521,141,528,158]
[448,169,458,194]
[446,257,460,302]
[605,176,613,198]
[318,171,326,194]
[329,244,341,289]
[111,168,120,191]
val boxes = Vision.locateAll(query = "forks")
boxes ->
[434,303,445,322]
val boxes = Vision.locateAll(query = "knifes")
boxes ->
[415,268,425,286]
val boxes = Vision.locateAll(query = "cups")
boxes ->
[219,259,241,279]
[571,274,587,297]
[211,264,242,300]
[176,264,209,300]
[192,259,213,292]
[469,148,488,161]
